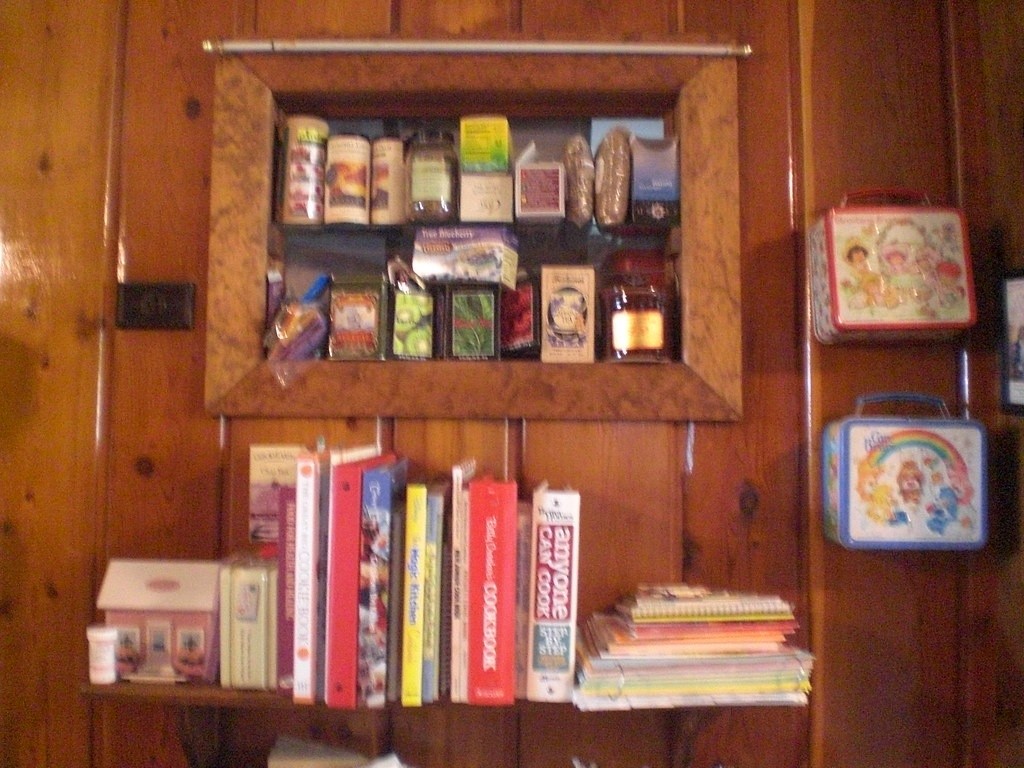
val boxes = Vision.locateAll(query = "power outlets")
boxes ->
[115,281,196,330]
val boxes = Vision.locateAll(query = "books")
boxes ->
[243,443,819,713]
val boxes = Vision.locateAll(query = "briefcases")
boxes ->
[823,389,987,552]
[809,183,976,345]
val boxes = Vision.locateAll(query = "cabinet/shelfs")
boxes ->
[200,35,755,422]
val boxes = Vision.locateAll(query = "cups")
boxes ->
[86,628,120,685]
